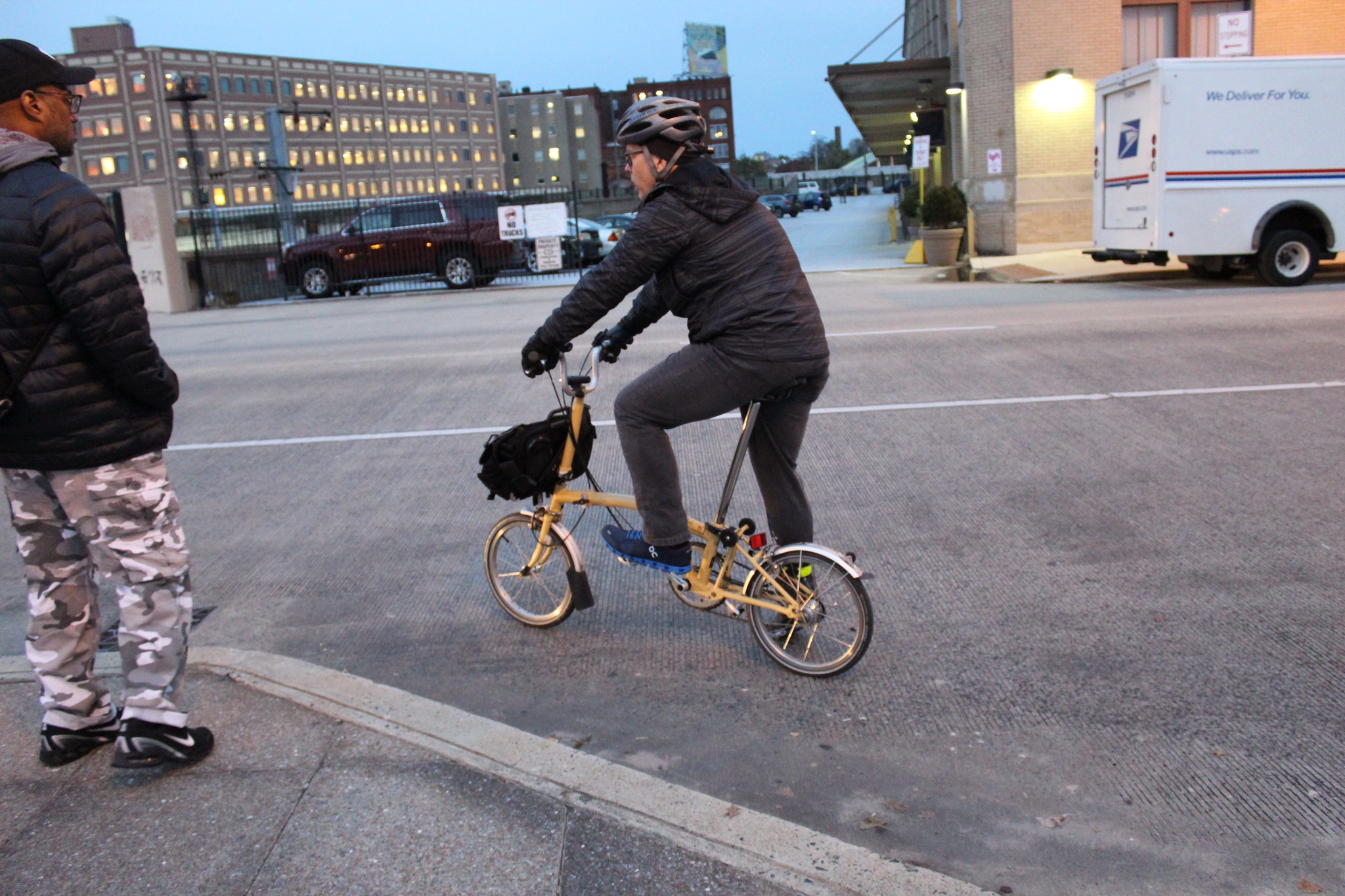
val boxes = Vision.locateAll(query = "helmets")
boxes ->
[616,96,715,157]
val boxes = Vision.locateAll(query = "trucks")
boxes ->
[1082,54,1345,284]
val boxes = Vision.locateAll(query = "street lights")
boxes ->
[811,130,818,179]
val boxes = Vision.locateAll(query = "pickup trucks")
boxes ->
[280,192,528,299]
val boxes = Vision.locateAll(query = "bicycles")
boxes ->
[484,325,875,679]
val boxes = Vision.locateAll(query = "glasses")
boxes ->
[15,90,83,114]
[623,149,645,166]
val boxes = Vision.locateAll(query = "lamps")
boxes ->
[1044,68,1076,78]
[946,81,966,94]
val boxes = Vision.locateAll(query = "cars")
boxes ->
[758,177,909,219]
[508,210,640,275]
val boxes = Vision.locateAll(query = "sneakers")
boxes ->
[111,717,215,769]
[39,706,125,766]
[774,565,818,601]
[601,523,693,574]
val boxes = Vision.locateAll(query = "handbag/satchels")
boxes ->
[478,404,598,501]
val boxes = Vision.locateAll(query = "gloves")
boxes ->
[597,322,628,363]
[522,325,561,379]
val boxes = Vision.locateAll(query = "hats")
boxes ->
[0,38,97,106]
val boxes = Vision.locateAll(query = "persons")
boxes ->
[0,36,216,774]
[516,94,829,636]
[896,171,910,179]
[818,176,831,195]
[851,174,862,195]
[834,176,847,204]
[891,171,895,193]
[820,193,831,210]
[880,171,885,189]
[864,172,871,186]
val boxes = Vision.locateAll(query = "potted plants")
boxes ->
[899,180,967,267]
